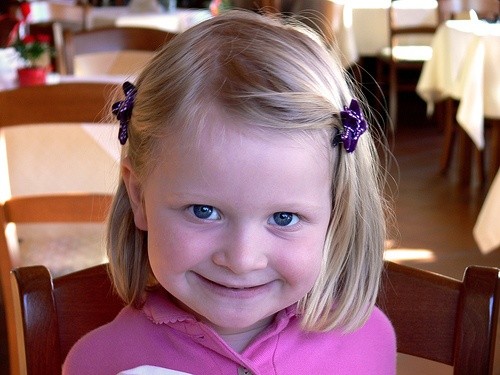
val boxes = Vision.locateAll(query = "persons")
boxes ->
[62,10,396,375]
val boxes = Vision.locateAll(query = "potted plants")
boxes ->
[13,34,60,86]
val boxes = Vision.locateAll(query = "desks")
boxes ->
[437,20,500,195]
[301,0,438,125]
[0,124,121,276]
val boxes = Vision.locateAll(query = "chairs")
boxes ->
[0,0,500,375]
